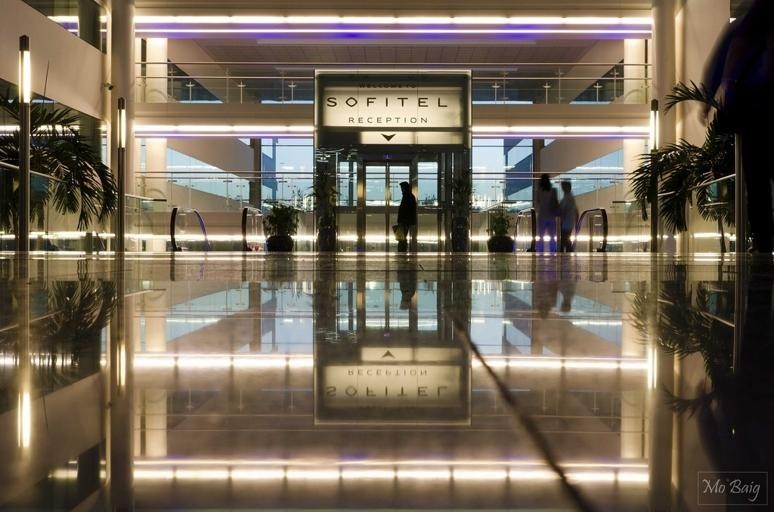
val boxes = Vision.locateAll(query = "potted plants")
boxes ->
[449,166,516,252]
[261,164,338,252]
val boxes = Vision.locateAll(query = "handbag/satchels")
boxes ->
[393,225,409,241]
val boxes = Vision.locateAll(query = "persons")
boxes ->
[557,254,580,315]
[534,255,556,317]
[396,181,416,252]
[695,2,774,256]
[695,260,773,512]
[533,174,558,253]
[395,254,418,311]
[558,180,578,252]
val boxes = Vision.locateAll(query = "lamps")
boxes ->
[650,99,659,252]
[116,97,126,252]
[18,35,32,254]
